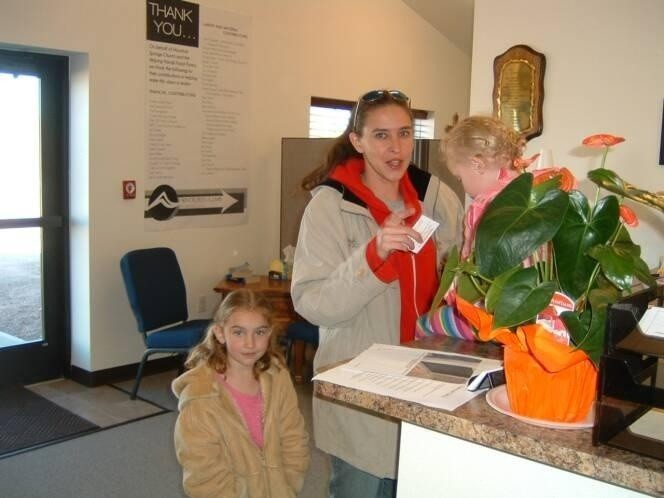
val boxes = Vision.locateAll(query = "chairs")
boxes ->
[119,247,217,399]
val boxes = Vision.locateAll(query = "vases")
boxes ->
[493,307,599,423]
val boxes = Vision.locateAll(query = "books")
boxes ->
[226,262,260,284]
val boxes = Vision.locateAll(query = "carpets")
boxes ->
[0,387,102,456]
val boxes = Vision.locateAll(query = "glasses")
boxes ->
[353,89,411,133]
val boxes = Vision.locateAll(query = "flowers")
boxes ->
[429,130,664,363]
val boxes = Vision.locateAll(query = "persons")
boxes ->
[170,288,310,498]
[289,89,466,498]
[414,115,552,342]
